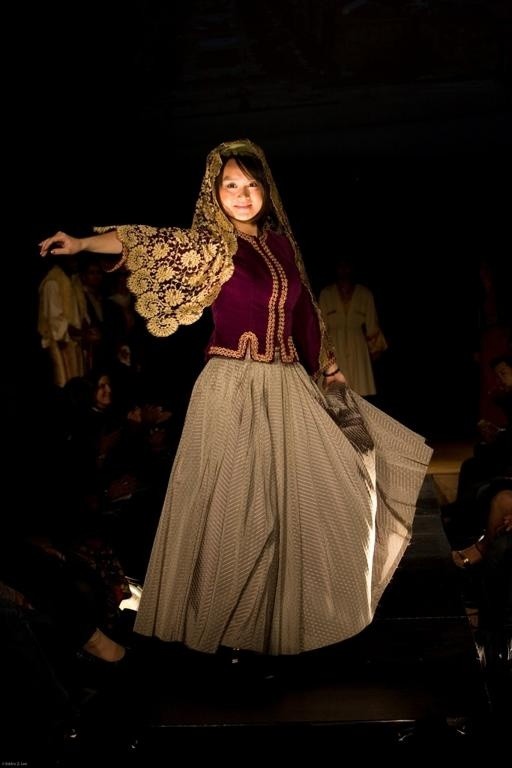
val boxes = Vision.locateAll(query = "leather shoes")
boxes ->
[81,647,136,668]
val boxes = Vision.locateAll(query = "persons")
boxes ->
[318,252,385,405]
[38,136,378,701]
[396,352,511,767]
[0,228,181,768]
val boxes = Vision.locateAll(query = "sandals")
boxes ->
[451,543,485,569]
